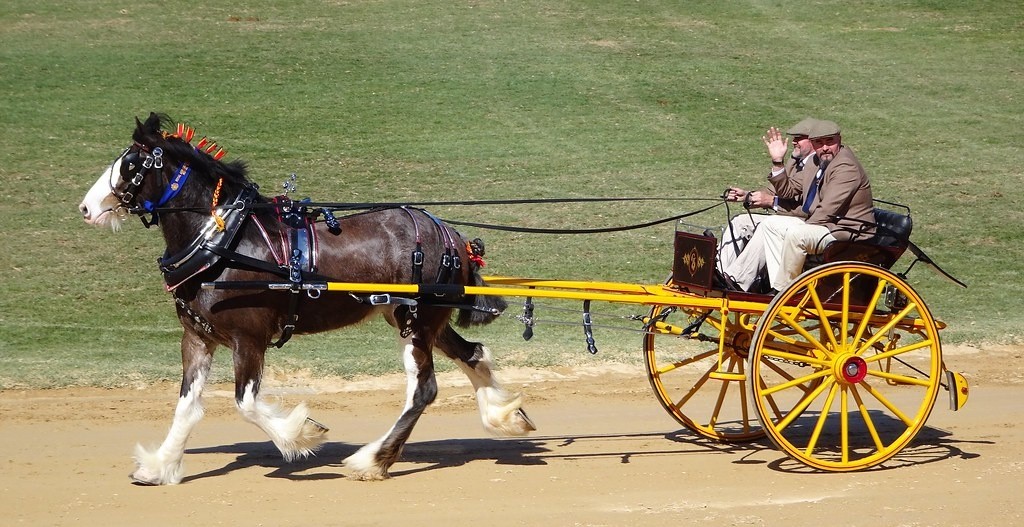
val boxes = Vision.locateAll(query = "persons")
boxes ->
[725,119,878,297]
[716,117,820,274]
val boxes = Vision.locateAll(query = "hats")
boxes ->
[786,118,823,137]
[808,120,841,140]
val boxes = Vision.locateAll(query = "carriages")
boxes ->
[77,111,972,486]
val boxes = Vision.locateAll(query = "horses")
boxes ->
[78,111,537,487]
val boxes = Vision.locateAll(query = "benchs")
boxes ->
[805,198,914,265]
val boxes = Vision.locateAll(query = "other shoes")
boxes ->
[767,289,779,295]
[723,272,742,291]
[713,272,726,288]
